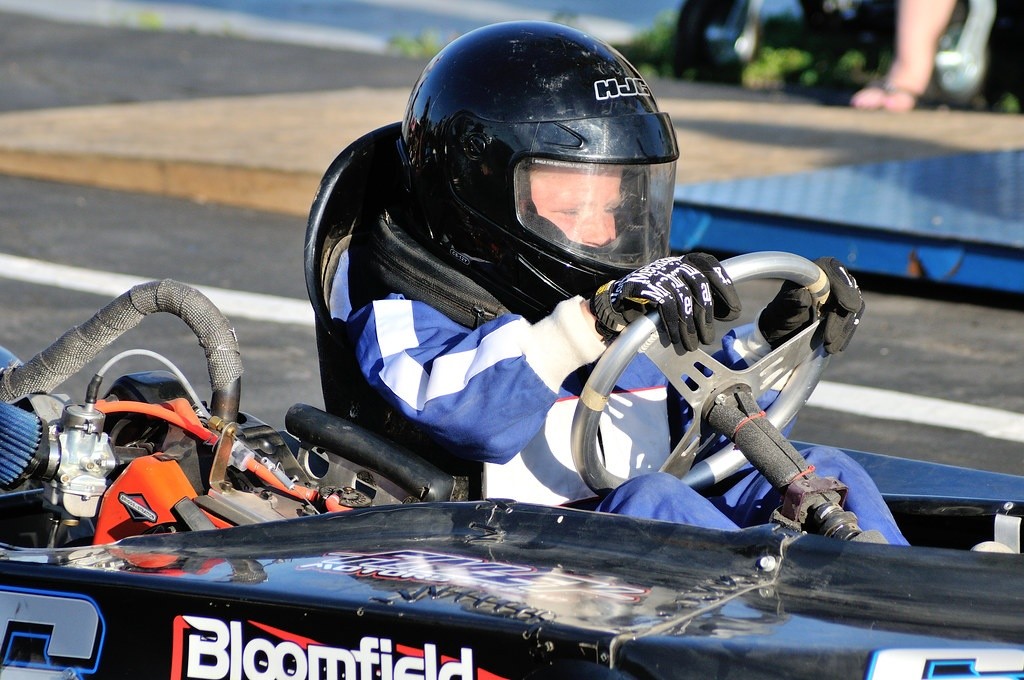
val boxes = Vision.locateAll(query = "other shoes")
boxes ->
[849,88,918,113]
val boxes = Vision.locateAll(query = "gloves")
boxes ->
[589,251,741,352]
[757,257,865,353]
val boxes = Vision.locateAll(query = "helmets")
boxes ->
[394,21,679,324]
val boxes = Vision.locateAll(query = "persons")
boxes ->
[322,22,914,546]
